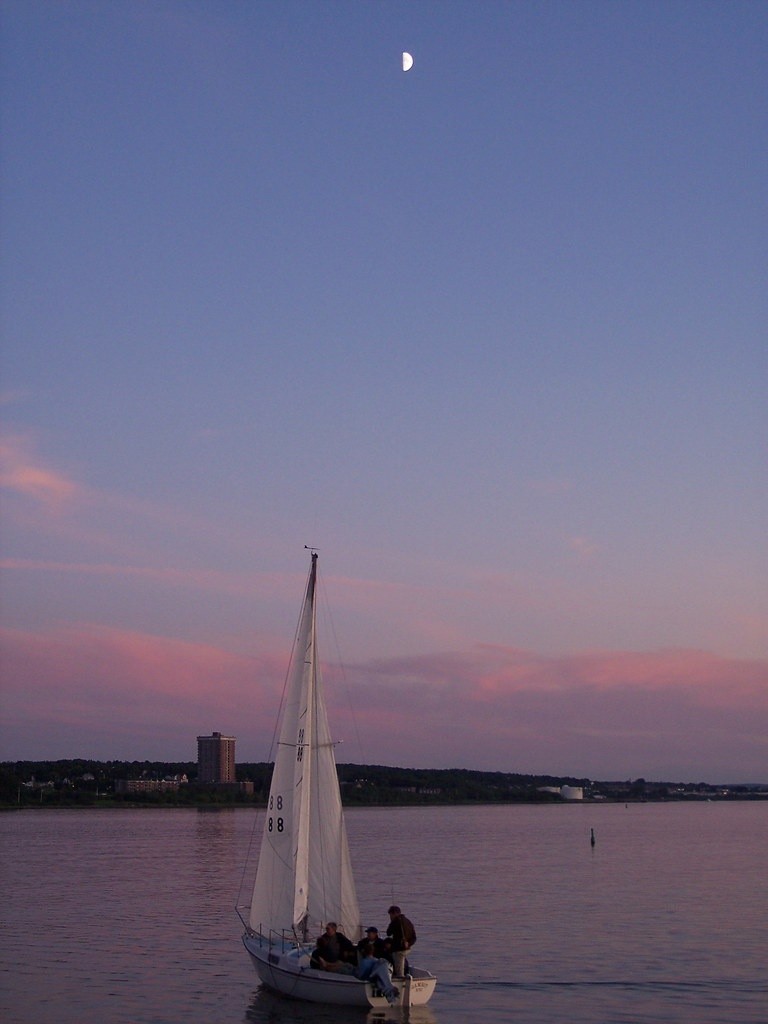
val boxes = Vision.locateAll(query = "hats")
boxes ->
[365,927,378,932]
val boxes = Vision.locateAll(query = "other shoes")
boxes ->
[385,987,399,1002]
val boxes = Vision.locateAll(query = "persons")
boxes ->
[387,906,416,979]
[311,922,409,1005]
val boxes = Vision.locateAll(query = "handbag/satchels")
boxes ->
[400,938,409,950]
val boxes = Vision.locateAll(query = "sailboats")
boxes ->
[232,545,438,1009]
